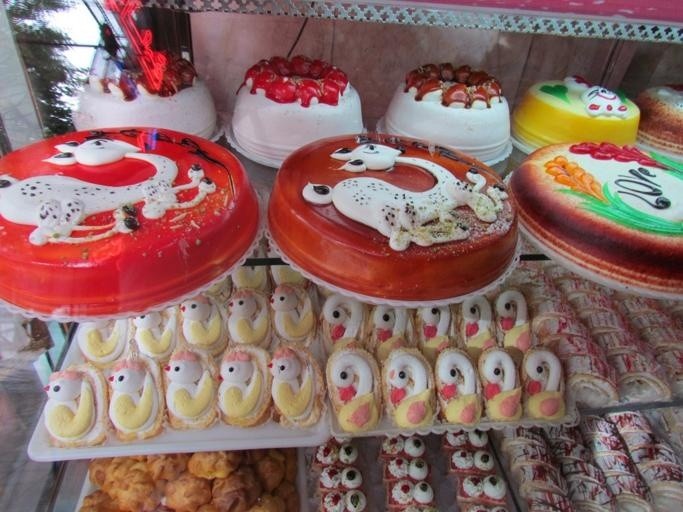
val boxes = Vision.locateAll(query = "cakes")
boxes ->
[73,50,217,141]
[510,74,641,152]
[267,132,518,301]
[384,62,510,163]
[231,54,364,160]
[508,139,683,292]
[631,83,683,145]
[0,126,260,318]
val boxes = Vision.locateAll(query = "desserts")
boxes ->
[41,237,683,512]
